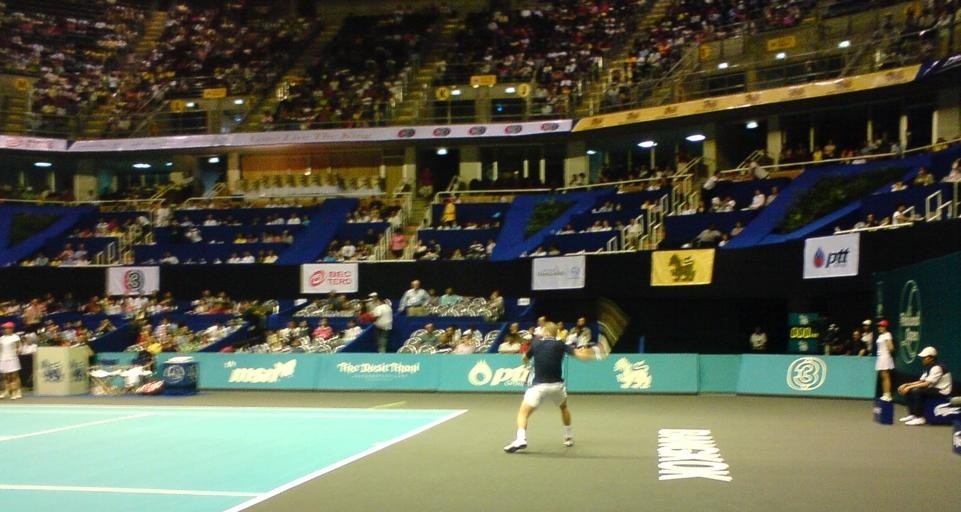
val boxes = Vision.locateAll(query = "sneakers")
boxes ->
[563,439,572,446]
[899,415,925,425]
[504,441,527,452]
[880,395,893,401]
[1,393,22,400]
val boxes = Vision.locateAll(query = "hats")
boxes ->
[877,320,888,326]
[918,347,938,358]
[2,322,16,328]
[862,319,872,326]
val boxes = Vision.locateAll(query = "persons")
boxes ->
[1,1,961,139]
[0,321,22,399]
[1,280,505,396]
[820,320,873,355]
[897,347,952,426]
[423,132,961,259]
[498,317,599,355]
[1,165,433,267]
[503,321,584,453]
[875,319,895,401]
[750,325,767,354]
